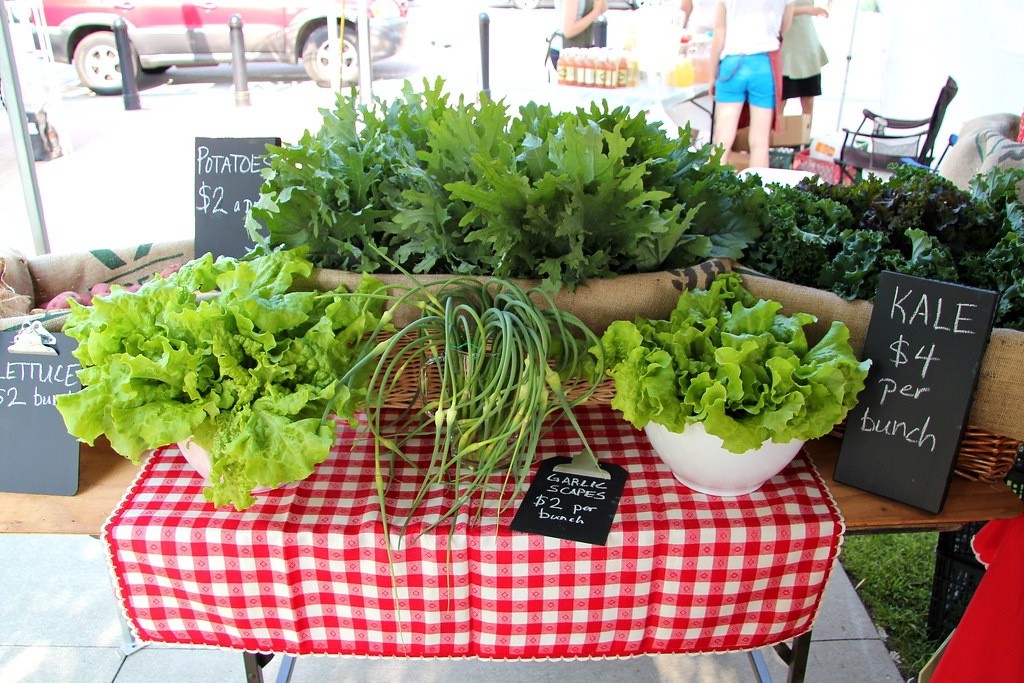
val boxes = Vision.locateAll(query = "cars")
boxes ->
[487,0,664,10]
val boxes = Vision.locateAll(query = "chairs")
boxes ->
[834,74,959,185]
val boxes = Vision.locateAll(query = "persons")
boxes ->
[781,0,829,115]
[709,0,794,167]
[681,0,713,55]
[563,0,609,48]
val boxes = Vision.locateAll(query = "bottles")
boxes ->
[556,47,639,89]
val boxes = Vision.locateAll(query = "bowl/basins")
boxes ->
[642,413,813,497]
[175,433,295,494]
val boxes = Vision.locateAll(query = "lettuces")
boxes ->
[55,247,389,510]
[595,270,874,453]
[745,158,1024,331]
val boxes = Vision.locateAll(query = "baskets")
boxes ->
[730,263,1024,485]
[284,254,736,407]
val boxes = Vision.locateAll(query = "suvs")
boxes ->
[8,0,408,96]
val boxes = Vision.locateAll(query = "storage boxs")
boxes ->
[734,114,855,186]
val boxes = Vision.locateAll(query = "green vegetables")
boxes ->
[254,77,763,295]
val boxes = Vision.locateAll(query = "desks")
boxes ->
[549,76,715,154]
[1,433,1024,683]
